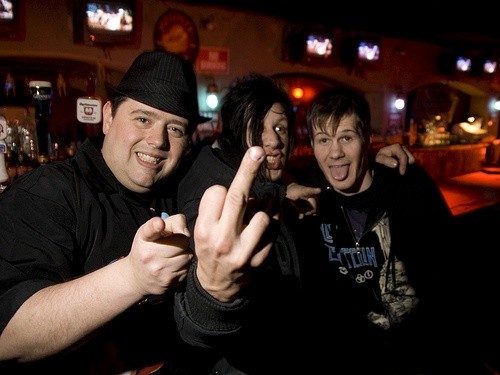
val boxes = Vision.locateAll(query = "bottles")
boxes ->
[75,72,102,142]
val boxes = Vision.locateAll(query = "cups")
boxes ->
[49,142,63,161]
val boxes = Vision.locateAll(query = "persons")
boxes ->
[173,71,461,375]
[0,48,212,375]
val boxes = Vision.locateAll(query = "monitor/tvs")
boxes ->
[290,29,336,66]
[340,35,382,67]
[437,53,474,75]
[73,0,144,49]
[0,0,26,42]
[475,57,498,76]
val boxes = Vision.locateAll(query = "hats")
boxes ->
[105,50,212,125]
